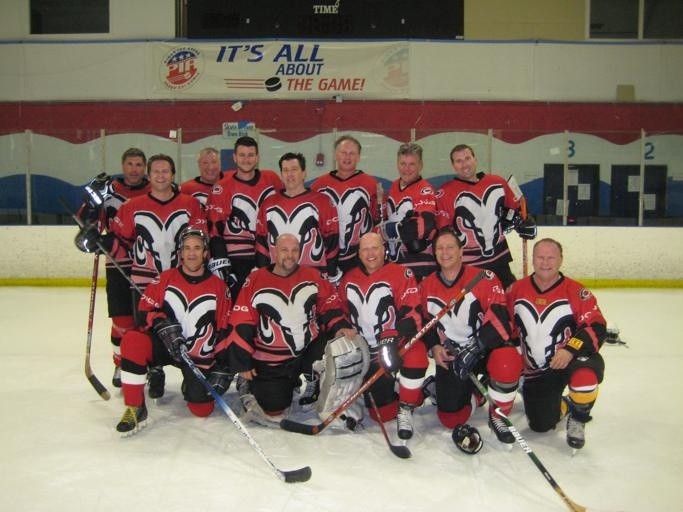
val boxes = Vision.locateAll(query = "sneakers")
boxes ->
[297,378,321,407]
[116,404,149,439]
[487,410,517,454]
[565,412,587,460]
[395,403,414,446]
[148,367,166,406]
[112,365,122,388]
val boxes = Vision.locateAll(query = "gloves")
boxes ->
[513,214,541,239]
[452,342,482,380]
[375,329,401,375]
[82,171,117,208]
[204,368,235,398]
[74,223,101,254]
[154,322,187,364]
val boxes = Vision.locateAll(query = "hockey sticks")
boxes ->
[278,270,488,435]
[84,251,113,402]
[177,346,311,483]
[367,389,411,459]
[445,338,588,512]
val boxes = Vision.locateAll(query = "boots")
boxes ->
[476,376,487,407]
[423,375,437,407]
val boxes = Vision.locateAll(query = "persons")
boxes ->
[76,148,152,387]
[229,234,355,418]
[116,226,232,437]
[206,137,285,303]
[254,151,340,277]
[419,227,522,444]
[310,137,386,279]
[436,145,537,287]
[384,143,437,283]
[77,152,212,399]
[335,231,429,439]
[179,147,225,208]
[507,238,607,447]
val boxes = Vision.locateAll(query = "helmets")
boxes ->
[178,224,211,248]
